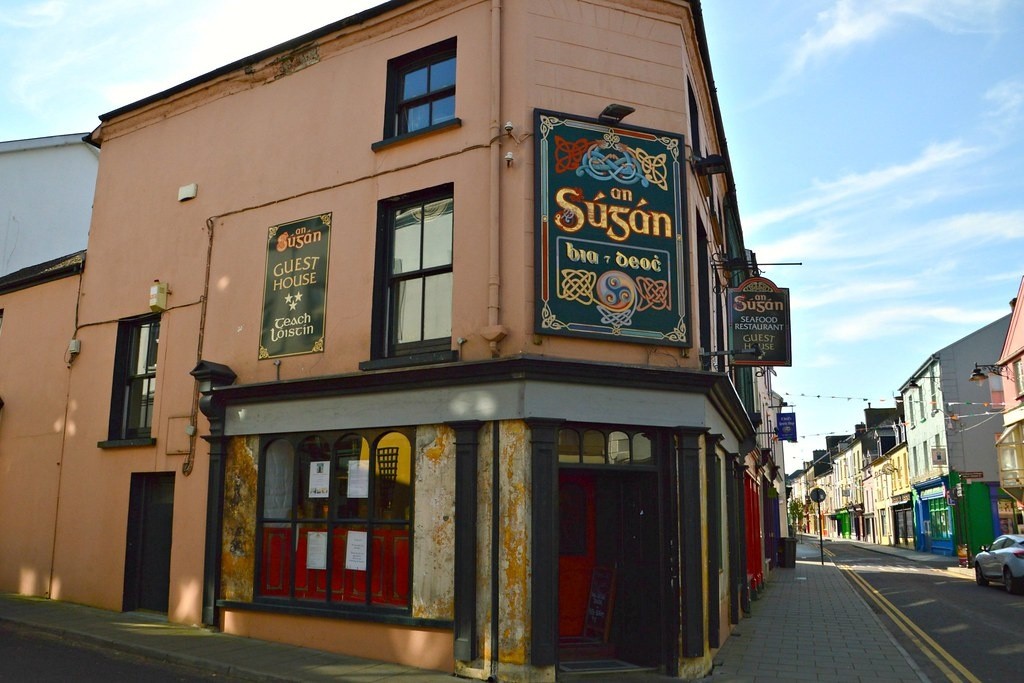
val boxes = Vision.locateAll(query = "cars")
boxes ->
[974,534,1024,594]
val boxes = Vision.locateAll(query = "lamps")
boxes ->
[692,154,729,175]
[969,362,1006,381]
[600,103,635,124]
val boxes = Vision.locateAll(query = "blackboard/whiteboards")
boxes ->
[585,565,619,629]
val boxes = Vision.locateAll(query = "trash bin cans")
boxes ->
[778,537,799,569]
